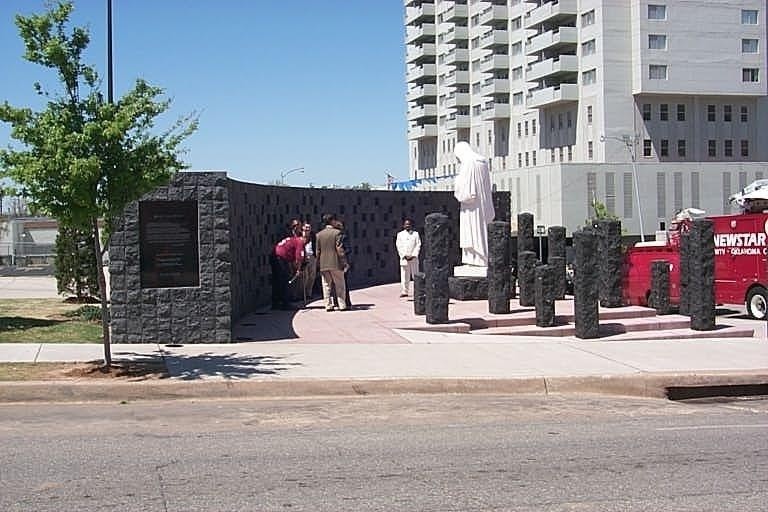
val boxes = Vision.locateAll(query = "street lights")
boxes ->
[600,133,645,244]
[281,162,305,188]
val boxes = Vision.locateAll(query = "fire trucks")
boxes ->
[616,212,768,321]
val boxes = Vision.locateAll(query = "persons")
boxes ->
[396,220,422,301]
[452,141,496,265]
[270,215,351,311]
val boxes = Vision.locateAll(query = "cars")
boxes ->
[559,246,576,296]
